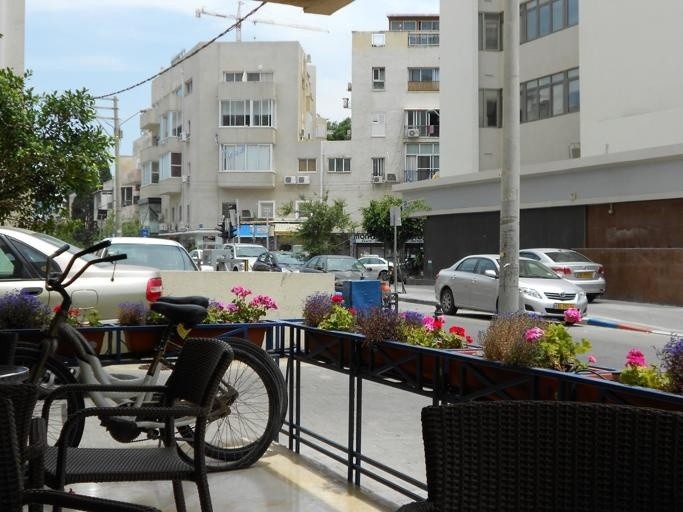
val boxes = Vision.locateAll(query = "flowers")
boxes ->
[207,283,278,326]
[0,286,79,331]
[517,306,670,391]
[304,292,474,354]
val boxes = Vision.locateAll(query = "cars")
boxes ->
[92,237,197,270]
[298,253,369,291]
[518,246,607,304]
[1,226,162,322]
[189,243,269,272]
[355,254,394,282]
[434,254,588,326]
[252,251,304,272]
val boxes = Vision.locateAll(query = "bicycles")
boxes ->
[2,239,289,472]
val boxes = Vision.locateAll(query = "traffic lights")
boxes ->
[215,222,225,239]
[230,221,238,239]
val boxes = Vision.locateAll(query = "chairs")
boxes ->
[3,335,43,511]
[41,338,232,510]
[390,399,683,512]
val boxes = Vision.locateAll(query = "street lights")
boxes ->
[114,109,148,237]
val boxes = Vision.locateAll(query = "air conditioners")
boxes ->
[407,128,420,137]
[284,176,309,185]
[373,172,400,184]
[241,209,254,218]
